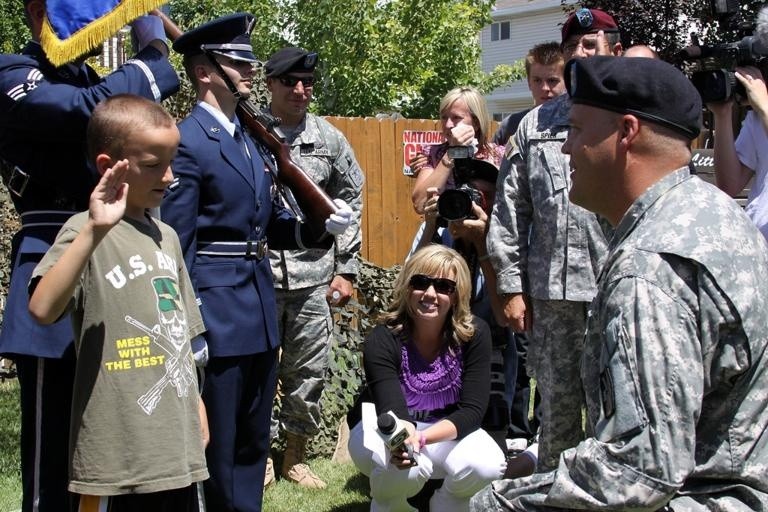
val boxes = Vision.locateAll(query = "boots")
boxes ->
[281,435,326,489]
[264,452,276,487]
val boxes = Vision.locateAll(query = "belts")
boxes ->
[195,239,270,261]
[20,208,78,226]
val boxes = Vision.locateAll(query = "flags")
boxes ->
[35,1,166,68]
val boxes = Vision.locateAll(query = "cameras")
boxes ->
[436,146,482,226]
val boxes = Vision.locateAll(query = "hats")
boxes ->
[172,11,257,63]
[563,56,703,139]
[264,46,318,76]
[454,156,499,184]
[562,8,617,41]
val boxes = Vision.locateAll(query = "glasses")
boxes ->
[410,274,456,294]
[280,74,317,87]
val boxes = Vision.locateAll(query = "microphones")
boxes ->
[376,411,417,473]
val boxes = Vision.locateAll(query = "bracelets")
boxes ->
[416,427,427,447]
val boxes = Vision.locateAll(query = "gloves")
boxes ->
[191,335,209,367]
[129,15,170,54]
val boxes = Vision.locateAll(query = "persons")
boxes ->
[403,158,519,456]
[411,80,508,217]
[490,40,568,144]
[26,94,211,512]
[485,9,621,470]
[259,49,364,489]
[624,42,659,63]
[0,1,183,511]
[346,243,506,512]
[466,56,767,512]
[709,8,767,242]
[164,10,354,511]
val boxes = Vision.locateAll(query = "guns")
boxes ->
[150,8,340,243]
